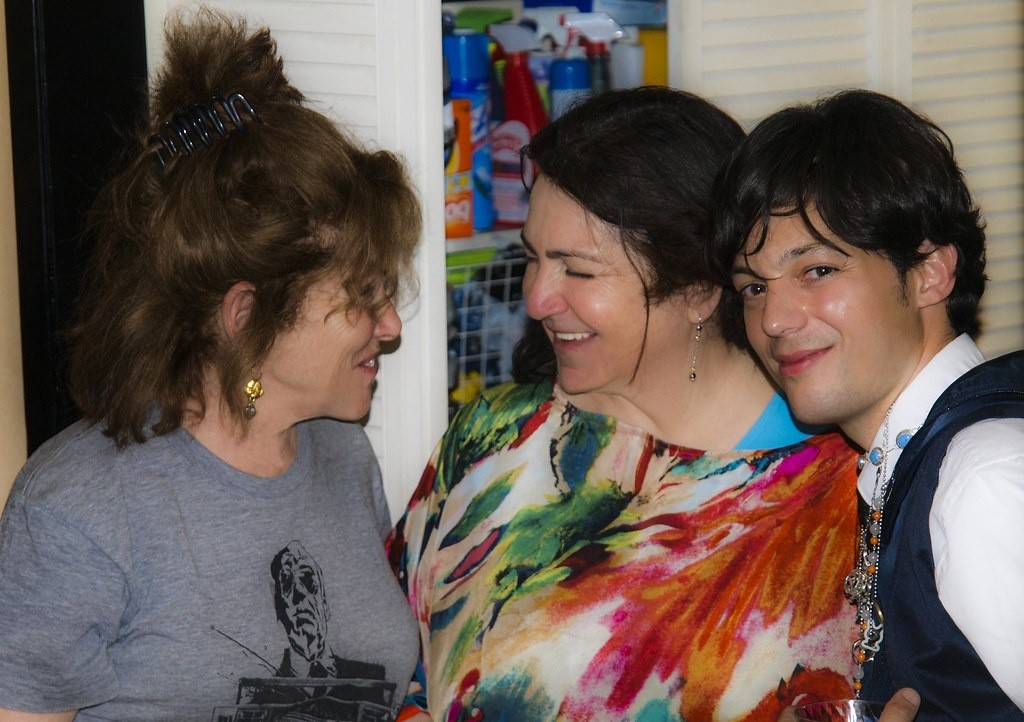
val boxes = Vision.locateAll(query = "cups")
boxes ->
[792,700,891,722]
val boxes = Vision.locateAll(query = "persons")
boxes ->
[0,23,422,722]
[384,85,867,722]
[709,90,1024,722]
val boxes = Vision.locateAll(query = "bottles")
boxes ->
[443,27,616,237]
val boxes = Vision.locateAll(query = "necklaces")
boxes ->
[843,401,890,700]
